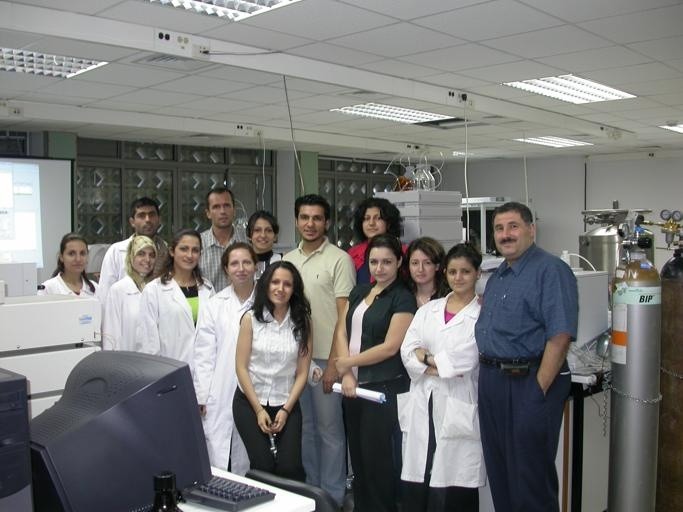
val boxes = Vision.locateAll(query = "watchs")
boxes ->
[424,351,432,366]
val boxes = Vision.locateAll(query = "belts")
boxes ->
[479,353,541,368]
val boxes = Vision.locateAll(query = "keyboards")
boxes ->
[185,476,276,512]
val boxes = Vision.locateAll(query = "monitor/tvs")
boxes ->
[28,349,214,512]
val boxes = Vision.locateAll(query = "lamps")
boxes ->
[329,102,462,128]
[0,48,111,80]
[150,0,307,25]
[511,135,597,151]
[501,74,640,105]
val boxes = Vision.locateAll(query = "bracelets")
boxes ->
[280,407,290,414]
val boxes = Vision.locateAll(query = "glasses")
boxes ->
[251,226,275,235]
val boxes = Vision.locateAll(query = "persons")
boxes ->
[98,196,168,287]
[134,229,216,380]
[197,188,252,293]
[333,233,417,512]
[192,242,258,476]
[102,236,158,351]
[232,260,314,482]
[348,197,410,285]
[396,243,487,512]
[474,202,580,512]
[282,194,357,511]
[402,237,452,309]
[37,233,99,298]
[246,210,283,283]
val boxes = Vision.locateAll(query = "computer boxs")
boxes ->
[0,368,32,497]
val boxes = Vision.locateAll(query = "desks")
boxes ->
[1,463,318,511]
